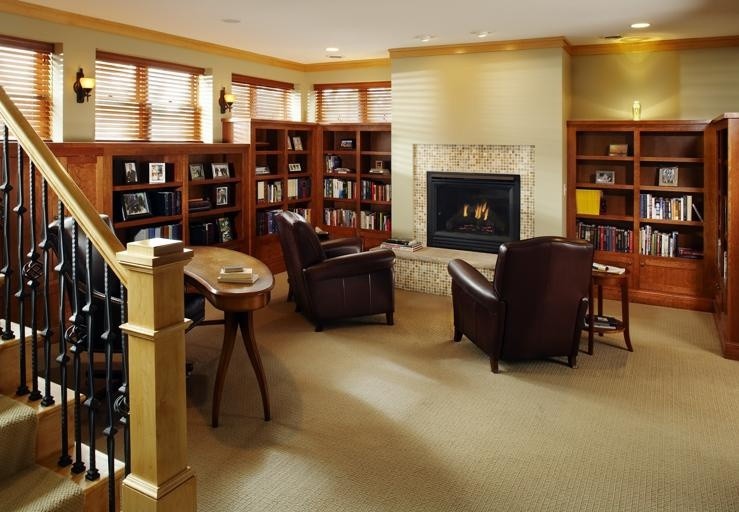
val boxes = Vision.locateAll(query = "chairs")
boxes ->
[272,210,395,332]
[43,213,205,425]
[447,235,593,373]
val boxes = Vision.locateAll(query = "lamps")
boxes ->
[73,68,95,103]
[219,87,236,113]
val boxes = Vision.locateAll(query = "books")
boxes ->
[109,128,388,250]
[575,219,705,259]
[637,192,704,221]
[379,237,424,253]
[217,264,260,285]
[593,263,626,276]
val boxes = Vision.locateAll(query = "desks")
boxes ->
[183,246,275,428]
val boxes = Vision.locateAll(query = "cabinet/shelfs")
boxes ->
[315,123,393,255]
[3,140,252,340]
[223,116,314,282]
[566,112,739,361]
